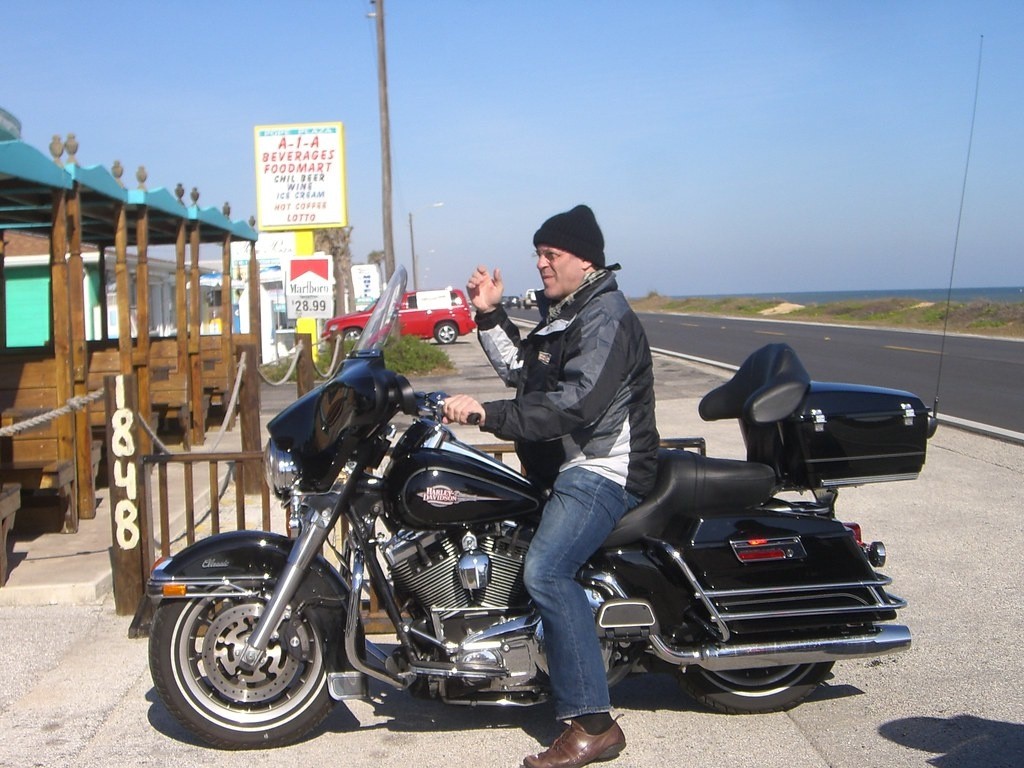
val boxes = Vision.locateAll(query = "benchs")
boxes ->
[132,348,159,472]
[88,352,121,475]
[150,339,192,451]
[0,483,22,588]
[0,348,79,533]
[189,340,211,444]
[78,413,103,519]
[190,336,224,444]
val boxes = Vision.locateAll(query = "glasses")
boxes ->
[533,250,569,262]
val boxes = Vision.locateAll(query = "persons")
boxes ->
[442,204,659,768]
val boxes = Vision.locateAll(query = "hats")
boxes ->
[533,205,605,268]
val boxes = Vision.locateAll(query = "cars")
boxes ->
[499,288,543,309]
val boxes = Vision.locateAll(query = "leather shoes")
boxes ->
[524,714,626,768]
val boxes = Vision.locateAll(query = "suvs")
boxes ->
[320,286,479,346]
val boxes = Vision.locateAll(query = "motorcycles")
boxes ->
[126,262,943,752]
